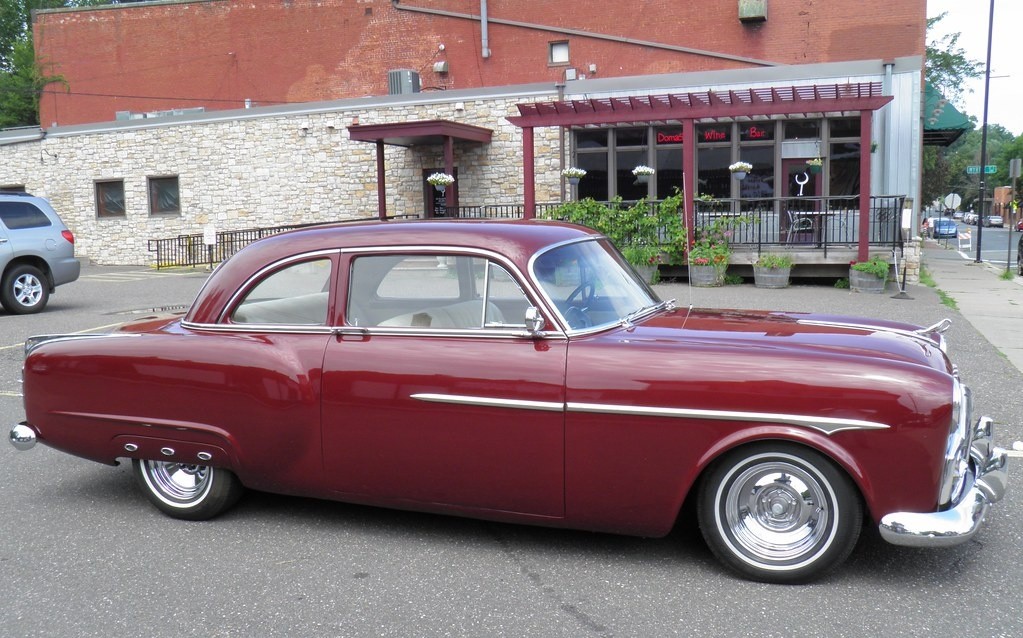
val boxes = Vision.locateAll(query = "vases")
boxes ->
[810,164,821,173]
[435,185,446,191]
[569,177,580,184]
[754,266,791,288]
[690,261,730,287]
[637,175,650,183]
[849,270,883,292]
[631,257,660,284]
[733,170,747,179]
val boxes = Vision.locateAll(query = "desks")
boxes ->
[794,210,841,246]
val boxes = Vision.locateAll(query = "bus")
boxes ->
[935,197,944,212]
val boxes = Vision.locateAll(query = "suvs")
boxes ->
[0,191,81,315]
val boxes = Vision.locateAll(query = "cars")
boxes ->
[952,211,1004,228]
[9,221,1008,585]
[933,219,959,239]
[944,208,955,216]
[1014,217,1023,275]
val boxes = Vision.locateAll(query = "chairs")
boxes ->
[784,210,819,246]
[450,298,510,331]
[379,309,455,329]
[820,208,850,243]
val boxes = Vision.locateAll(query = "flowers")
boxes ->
[806,159,824,165]
[622,247,663,263]
[754,254,796,270]
[428,172,454,185]
[561,167,588,178]
[632,165,655,175]
[689,244,733,266]
[850,257,889,274]
[729,162,753,173]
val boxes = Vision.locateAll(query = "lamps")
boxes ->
[302,122,310,130]
[326,119,334,127]
[40,149,58,165]
[455,102,464,111]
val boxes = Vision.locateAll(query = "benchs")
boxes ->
[246,292,356,322]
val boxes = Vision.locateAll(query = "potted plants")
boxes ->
[870,140,879,153]
